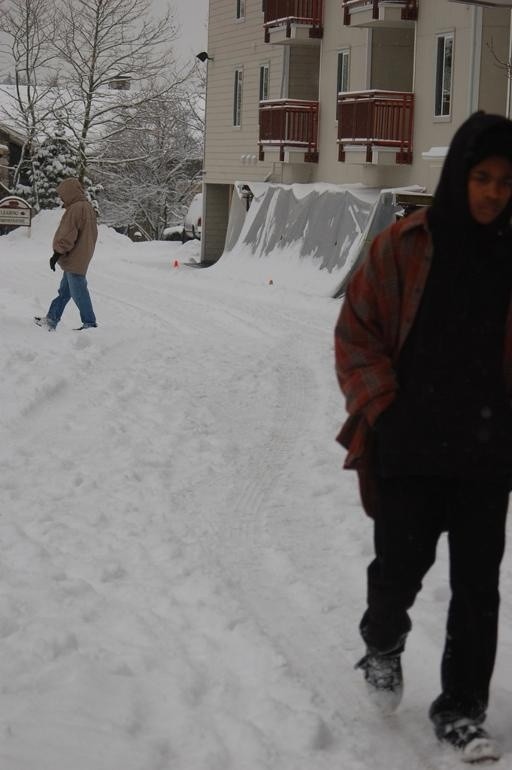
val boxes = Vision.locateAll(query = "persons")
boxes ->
[32,177,98,331]
[328,109,512,763]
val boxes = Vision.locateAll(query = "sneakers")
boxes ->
[33,316,57,333]
[434,718,501,763]
[353,642,403,713]
[73,324,97,330]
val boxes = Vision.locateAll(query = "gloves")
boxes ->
[50,252,61,271]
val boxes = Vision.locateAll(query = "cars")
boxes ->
[163,226,184,240]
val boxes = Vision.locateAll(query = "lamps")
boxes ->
[195,51,213,63]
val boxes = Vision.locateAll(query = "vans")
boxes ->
[185,193,202,237]
